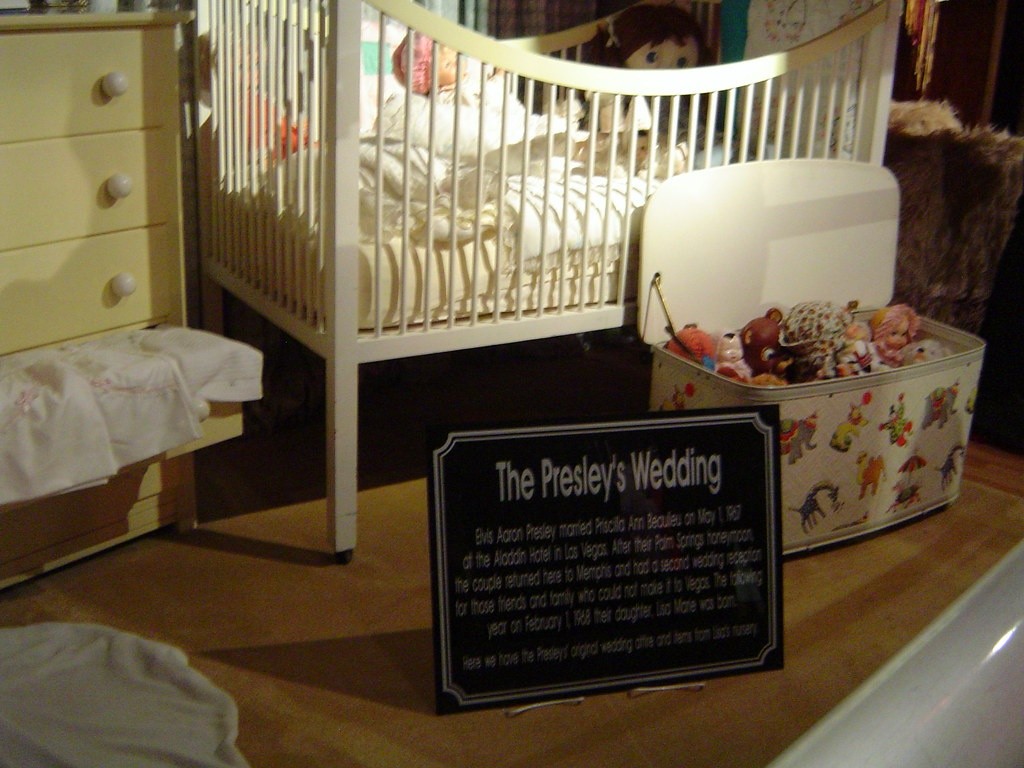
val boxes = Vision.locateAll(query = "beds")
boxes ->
[190,0,903,565]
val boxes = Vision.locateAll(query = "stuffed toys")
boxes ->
[589,3,708,131]
[663,299,953,386]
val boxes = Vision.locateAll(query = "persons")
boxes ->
[391,30,575,135]
[198,23,311,166]
[869,303,918,368]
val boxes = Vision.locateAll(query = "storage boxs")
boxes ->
[635,159,987,559]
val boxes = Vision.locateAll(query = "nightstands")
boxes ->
[0,10,263,592]
